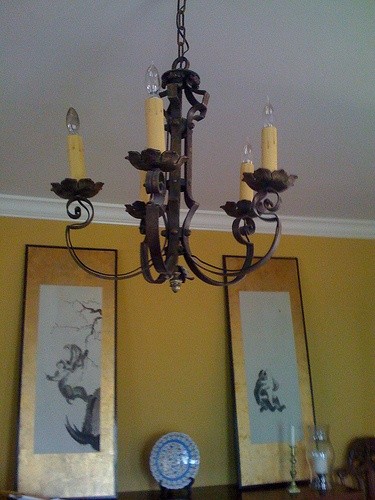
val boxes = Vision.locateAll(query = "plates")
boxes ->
[150,432,200,491]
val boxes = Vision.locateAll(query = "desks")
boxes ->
[123,479,363,500]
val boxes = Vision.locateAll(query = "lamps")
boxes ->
[46,2,298,292]
